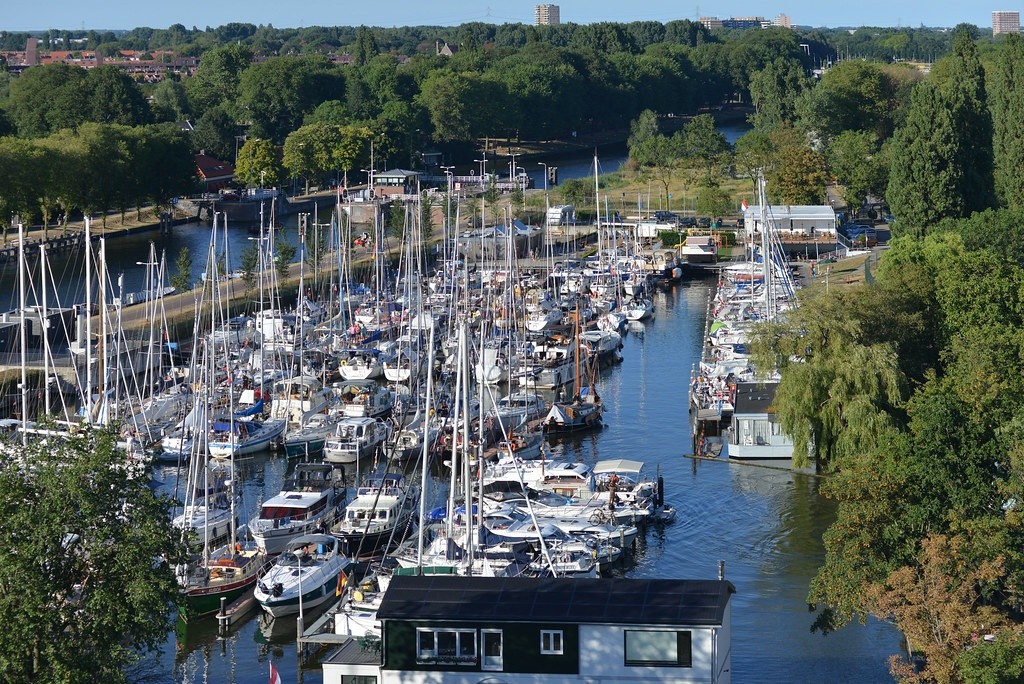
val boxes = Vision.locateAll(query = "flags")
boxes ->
[336,568,348,596]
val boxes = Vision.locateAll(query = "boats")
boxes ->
[457,480,651,527]
[422,504,478,522]
[255,589,340,664]
[253,533,354,617]
[543,460,592,496]
[249,462,348,555]
[507,520,620,566]
[483,506,638,549]
[329,471,421,544]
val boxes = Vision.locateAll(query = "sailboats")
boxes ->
[0,137,806,639]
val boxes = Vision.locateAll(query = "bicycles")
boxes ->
[593,505,617,521]
[589,513,618,531]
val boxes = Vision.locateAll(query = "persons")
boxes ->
[511,440,515,452]
[304,547,309,555]
[430,407,435,418]
[550,416,556,424]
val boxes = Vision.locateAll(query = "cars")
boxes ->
[699,217,713,228]
[883,215,896,223]
[219,194,241,201]
[847,225,878,241]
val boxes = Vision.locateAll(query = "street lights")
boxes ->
[360,169,377,202]
[249,140,259,196]
[833,181,837,189]
[440,166,455,181]
[508,162,511,182]
[411,129,420,170]
[507,153,521,182]
[538,162,547,194]
[371,133,385,189]
[294,144,306,197]
[473,160,488,186]
[444,171,453,199]
[517,167,525,195]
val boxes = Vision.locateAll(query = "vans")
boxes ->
[545,204,575,226]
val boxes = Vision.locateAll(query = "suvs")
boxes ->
[736,219,744,228]
[679,216,697,228]
[654,211,680,222]
[848,219,875,228]
